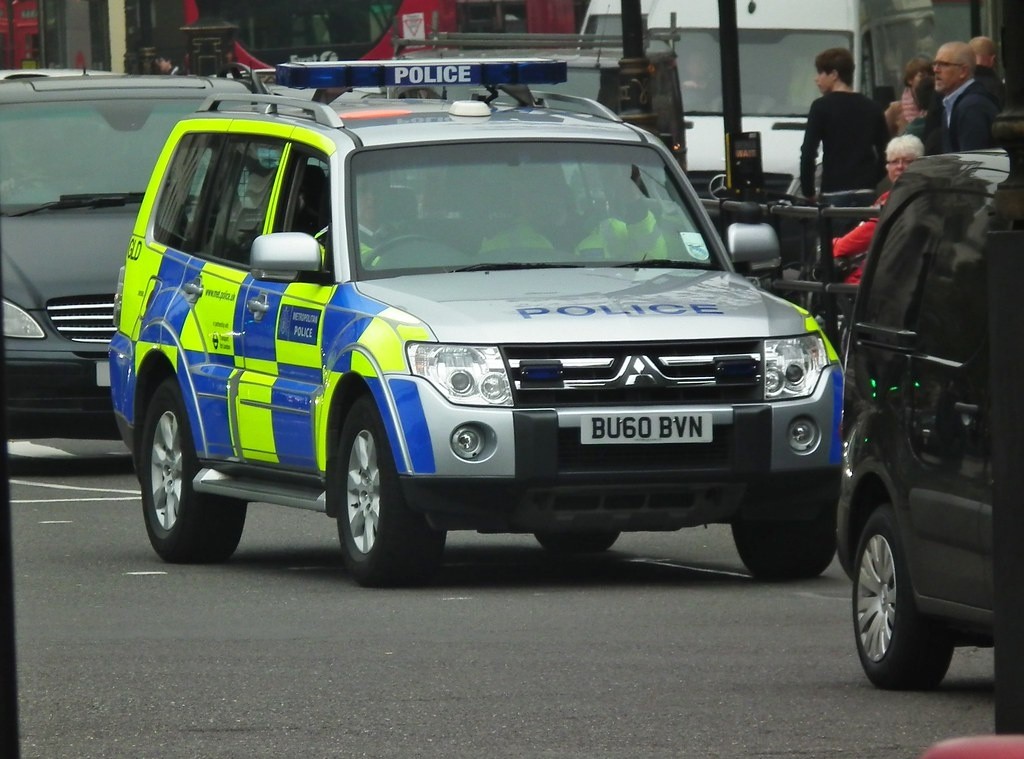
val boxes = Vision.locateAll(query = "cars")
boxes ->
[834,147,1020,686]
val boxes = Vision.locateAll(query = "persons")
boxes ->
[155,48,194,76]
[884,57,936,136]
[904,78,938,140]
[478,162,668,263]
[799,48,890,284]
[313,177,400,269]
[969,35,1006,110]
[922,42,995,156]
[832,133,925,284]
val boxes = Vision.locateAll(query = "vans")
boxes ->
[574,0,916,270]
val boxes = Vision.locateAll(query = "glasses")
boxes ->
[886,158,914,165]
[931,60,963,68]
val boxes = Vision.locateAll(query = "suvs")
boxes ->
[104,59,844,581]
[1,61,282,446]
